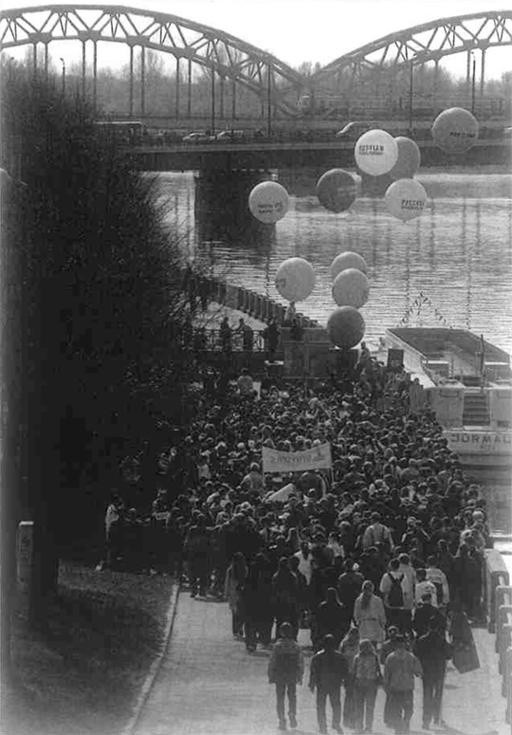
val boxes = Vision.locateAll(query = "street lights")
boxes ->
[469,48,477,115]
[410,49,416,134]
[59,56,67,99]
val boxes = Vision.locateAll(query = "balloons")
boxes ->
[316,129,426,221]
[275,252,371,348]
[430,106,479,151]
[249,182,290,224]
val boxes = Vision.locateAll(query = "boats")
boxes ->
[382,289,511,530]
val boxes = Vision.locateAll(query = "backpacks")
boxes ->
[387,572,405,607]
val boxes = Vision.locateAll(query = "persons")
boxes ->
[267,622,424,733]
[413,615,452,729]
[105,316,492,652]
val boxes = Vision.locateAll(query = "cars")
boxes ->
[84,119,383,142]
[503,123,511,138]
